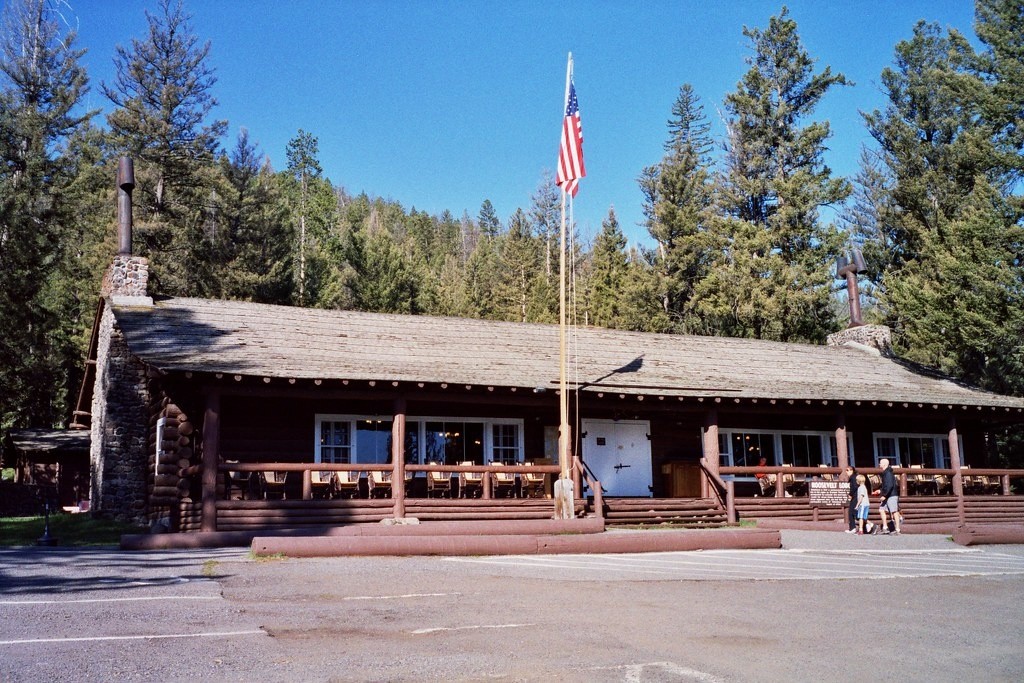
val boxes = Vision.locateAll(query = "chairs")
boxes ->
[222,459,550,498]
[758,463,1001,497]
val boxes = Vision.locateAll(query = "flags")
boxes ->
[555,81,586,198]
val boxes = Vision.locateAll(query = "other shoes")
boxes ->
[845,528,856,533]
[866,523,874,532]
[872,524,879,534]
[878,528,890,534]
[890,530,901,535]
[854,530,864,535]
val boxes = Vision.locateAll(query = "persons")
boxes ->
[854,475,878,535]
[844,467,873,533]
[756,457,793,497]
[874,459,901,535]
